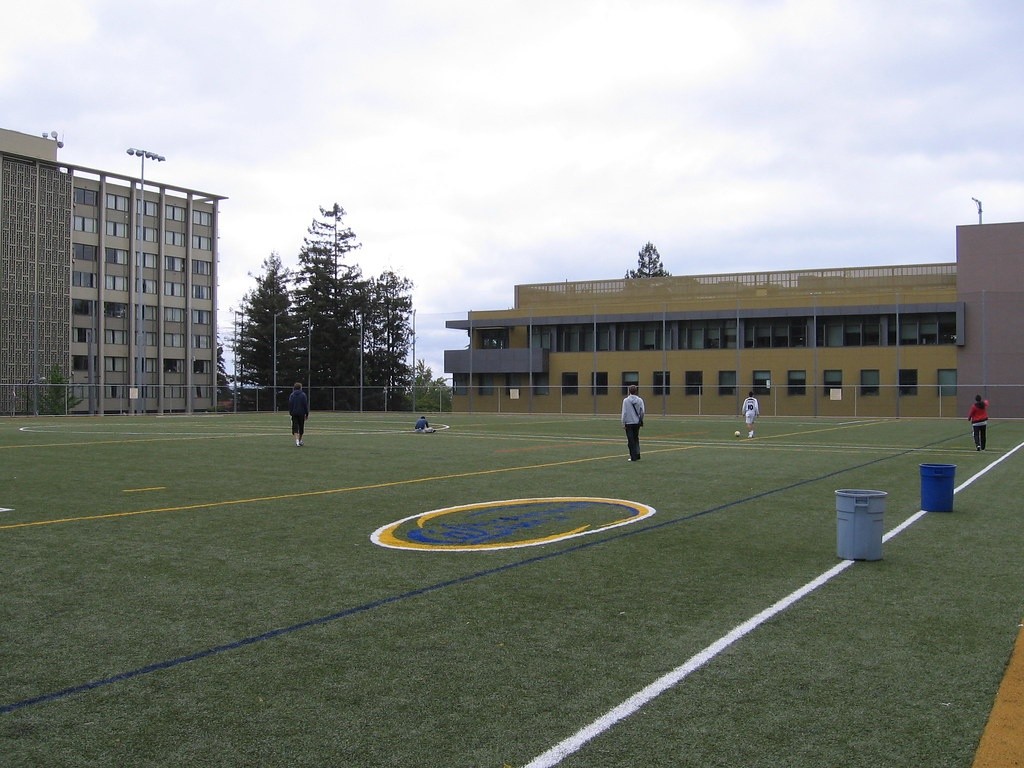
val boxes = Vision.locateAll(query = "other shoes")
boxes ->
[976,446,981,451]
[982,447,985,450]
[300,440,304,446]
[747,430,754,438]
[628,459,638,462]
[295,439,300,447]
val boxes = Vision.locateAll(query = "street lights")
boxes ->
[125,147,166,413]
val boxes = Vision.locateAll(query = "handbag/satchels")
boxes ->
[640,420,644,427]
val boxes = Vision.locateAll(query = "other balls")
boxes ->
[734,430,741,437]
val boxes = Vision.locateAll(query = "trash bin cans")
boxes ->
[835,489,888,561]
[919,463,957,512]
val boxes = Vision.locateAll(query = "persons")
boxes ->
[621,386,644,461]
[415,416,435,433]
[968,395,989,450]
[742,392,759,438]
[289,383,308,447]
